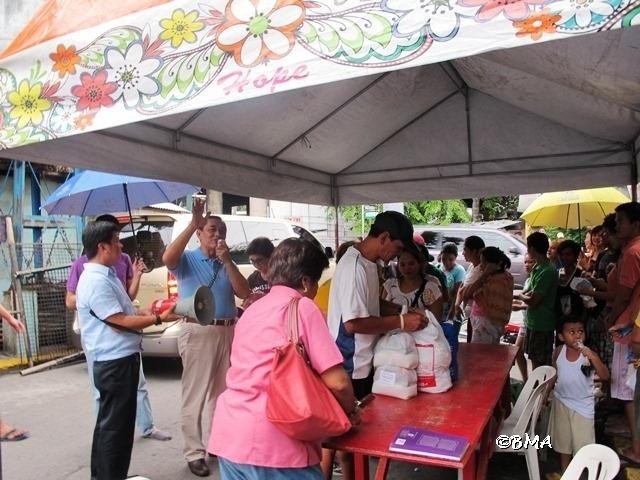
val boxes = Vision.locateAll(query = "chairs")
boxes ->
[457,365,557,480]
[560,444,620,480]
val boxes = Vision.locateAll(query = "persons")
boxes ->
[161,196,640,480]
[72,219,186,479]
[0,304,29,443]
[64,213,173,442]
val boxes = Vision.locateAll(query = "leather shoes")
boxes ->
[188,458,209,477]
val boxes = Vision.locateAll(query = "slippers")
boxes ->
[545,471,562,480]
[1,429,27,441]
[618,453,640,470]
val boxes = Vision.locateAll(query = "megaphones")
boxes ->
[152,285,216,326]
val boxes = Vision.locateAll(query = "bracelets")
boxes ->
[155,315,162,326]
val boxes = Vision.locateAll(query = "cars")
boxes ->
[412,223,532,293]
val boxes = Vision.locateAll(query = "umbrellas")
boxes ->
[37,169,203,274]
[517,186,633,249]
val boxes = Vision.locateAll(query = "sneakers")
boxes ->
[331,459,344,477]
[146,429,172,440]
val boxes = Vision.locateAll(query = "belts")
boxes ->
[183,316,236,325]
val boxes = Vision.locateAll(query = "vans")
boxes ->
[109,206,335,359]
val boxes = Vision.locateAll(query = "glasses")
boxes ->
[250,256,268,266]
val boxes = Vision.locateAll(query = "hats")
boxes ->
[375,211,422,254]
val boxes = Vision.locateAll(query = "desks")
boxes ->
[321,343,521,479]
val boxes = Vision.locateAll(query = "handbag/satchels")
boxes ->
[265,342,352,442]
